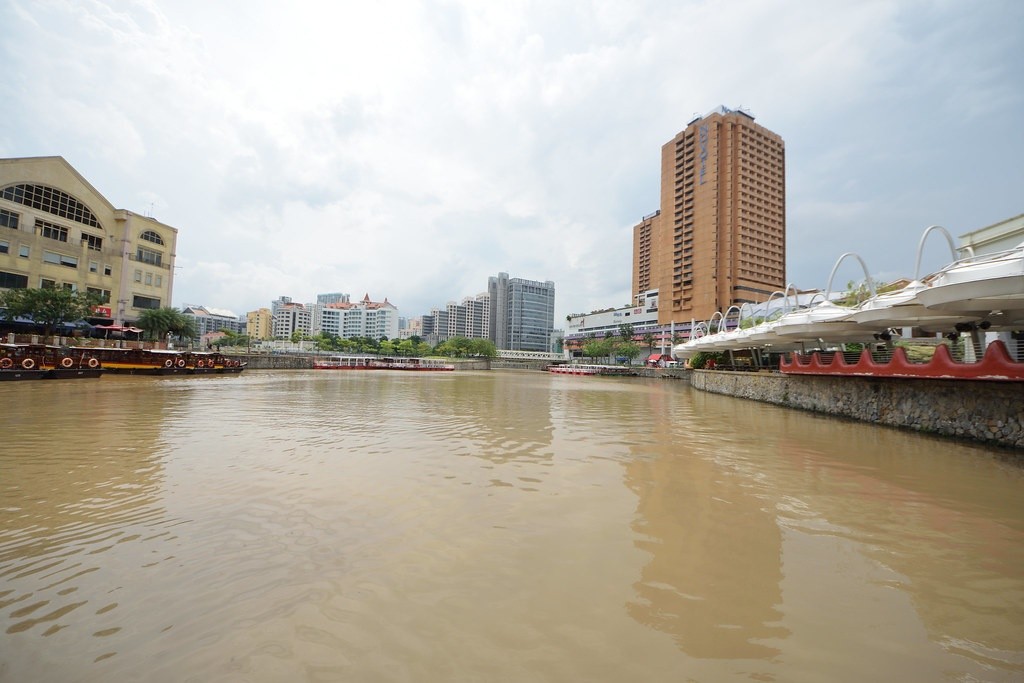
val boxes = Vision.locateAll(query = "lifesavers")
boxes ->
[227,361,231,366]
[165,359,172,367]
[235,361,239,367]
[22,358,35,369]
[178,359,185,367]
[88,358,98,367]
[208,360,214,367]
[62,357,74,367]
[0,357,13,368]
[198,360,204,367]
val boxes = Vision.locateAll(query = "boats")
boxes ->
[0,342,107,381]
[104,351,244,376]
[313,353,454,372]
[546,364,638,376]
